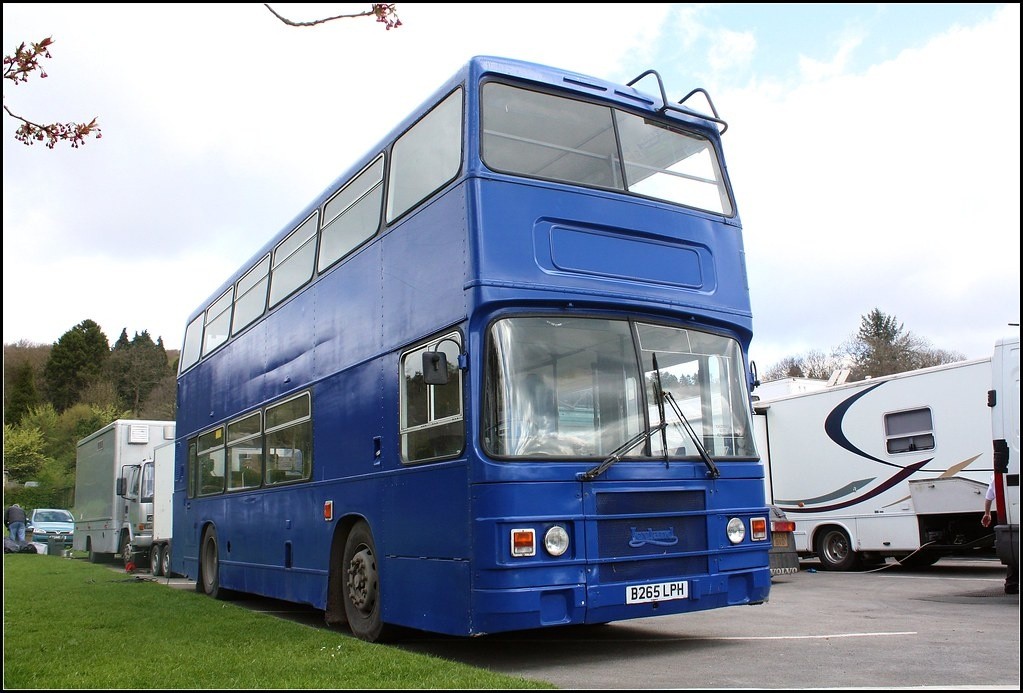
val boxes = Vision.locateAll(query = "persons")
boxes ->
[981,474,1018,594]
[5,504,26,542]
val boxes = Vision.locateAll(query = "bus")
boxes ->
[170,54,775,642]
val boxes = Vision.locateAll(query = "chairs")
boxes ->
[241,469,262,490]
[229,470,243,488]
[201,476,224,494]
[265,468,286,483]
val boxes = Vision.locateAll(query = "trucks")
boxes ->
[76,420,283,579]
[749,336,1018,596]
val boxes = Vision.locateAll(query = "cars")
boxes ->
[26,509,75,546]
